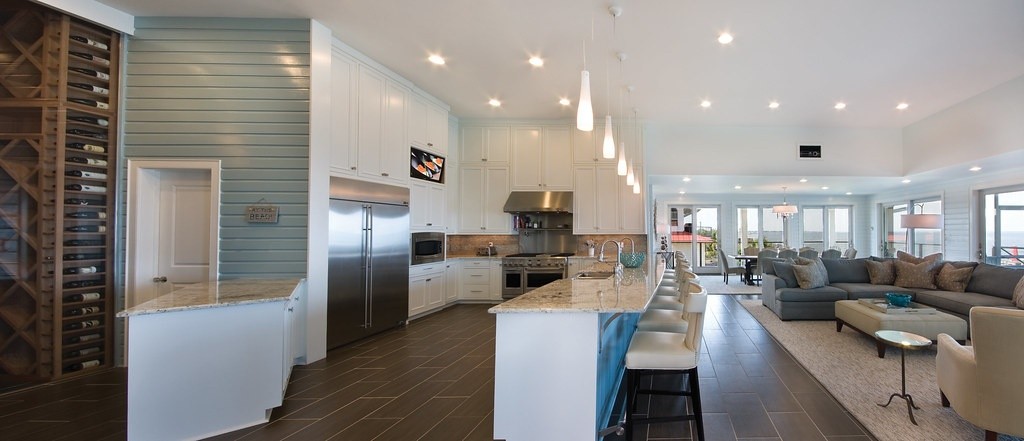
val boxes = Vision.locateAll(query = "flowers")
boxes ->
[584,239,598,249]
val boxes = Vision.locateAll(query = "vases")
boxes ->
[589,248,594,257]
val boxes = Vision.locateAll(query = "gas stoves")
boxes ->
[504,252,575,265]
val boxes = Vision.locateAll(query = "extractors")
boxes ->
[504,191,574,215]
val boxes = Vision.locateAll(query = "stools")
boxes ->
[624,251,709,441]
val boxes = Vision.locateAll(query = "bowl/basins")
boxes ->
[885,292,912,307]
[619,252,644,267]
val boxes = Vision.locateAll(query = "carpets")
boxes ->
[697,276,763,295]
[737,299,1024,441]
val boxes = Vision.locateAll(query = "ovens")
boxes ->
[411,231,446,266]
[501,256,568,300]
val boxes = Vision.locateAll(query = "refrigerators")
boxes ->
[327,175,409,351]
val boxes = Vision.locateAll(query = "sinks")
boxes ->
[574,278,608,279]
[578,272,614,277]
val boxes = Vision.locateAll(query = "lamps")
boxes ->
[772,186,798,220]
[634,106,641,195]
[602,5,622,160]
[576,39,594,132]
[900,203,942,230]
[615,52,627,175]
[624,88,635,187]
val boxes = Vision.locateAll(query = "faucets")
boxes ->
[620,237,635,255]
[597,239,624,278]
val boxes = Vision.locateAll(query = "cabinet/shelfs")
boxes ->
[37,9,119,380]
[409,259,502,320]
[116,278,306,441]
[568,258,597,278]
[330,47,647,236]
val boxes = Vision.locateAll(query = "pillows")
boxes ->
[771,250,974,293]
[1011,275,1024,309]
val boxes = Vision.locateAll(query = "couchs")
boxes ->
[759,256,1024,339]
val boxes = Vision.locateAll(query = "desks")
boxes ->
[873,330,932,425]
[727,254,849,286]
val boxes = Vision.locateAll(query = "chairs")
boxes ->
[718,246,858,288]
[934,305,1024,441]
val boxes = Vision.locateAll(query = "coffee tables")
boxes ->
[835,300,967,359]
